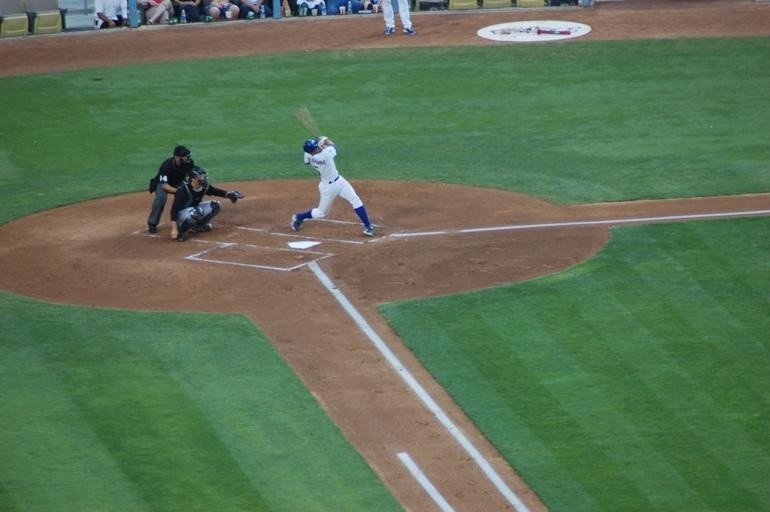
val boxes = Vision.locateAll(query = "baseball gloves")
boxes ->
[227,192,244,203]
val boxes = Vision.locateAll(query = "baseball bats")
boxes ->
[290,105,322,138]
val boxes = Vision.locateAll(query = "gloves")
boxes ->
[317,135,328,150]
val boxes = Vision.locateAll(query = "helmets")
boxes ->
[188,166,209,192]
[303,138,317,151]
[174,146,190,165]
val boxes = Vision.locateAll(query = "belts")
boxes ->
[328,173,340,185]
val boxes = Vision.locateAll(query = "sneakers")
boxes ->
[384,27,394,35]
[177,233,184,242]
[192,223,212,233]
[402,27,416,35]
[290,214,302,231]
[363,225,375,237]
[148,221,157,234]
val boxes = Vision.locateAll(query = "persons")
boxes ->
[147,144,195,233]
[169,166,245,242]
[290,135,375,237]
[93,1,417,35]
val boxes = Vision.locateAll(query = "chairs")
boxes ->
[0,0,67,37]
